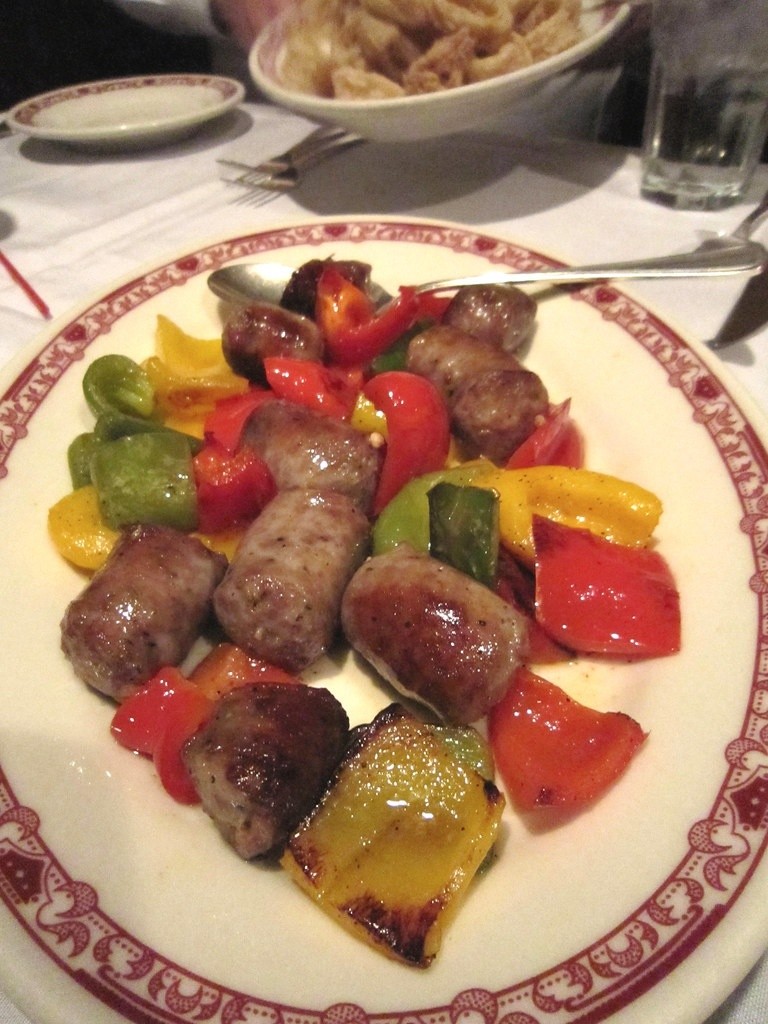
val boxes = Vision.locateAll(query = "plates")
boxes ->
[5,73,246,153]
[0,213,768,1024]
[248,0,632,144]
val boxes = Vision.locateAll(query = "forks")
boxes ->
[237,122,365,191]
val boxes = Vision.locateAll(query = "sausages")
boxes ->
[58,254,554,858]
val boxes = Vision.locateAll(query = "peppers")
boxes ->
[48,260,679,810]
[274,703,507,969]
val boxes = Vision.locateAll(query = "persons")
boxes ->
[101,0,652,145]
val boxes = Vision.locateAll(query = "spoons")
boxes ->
[206,242,764,311]
[694,189,768,254]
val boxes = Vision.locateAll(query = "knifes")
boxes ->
[708,268,768,349]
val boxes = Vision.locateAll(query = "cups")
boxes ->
[642,0,768,212]
